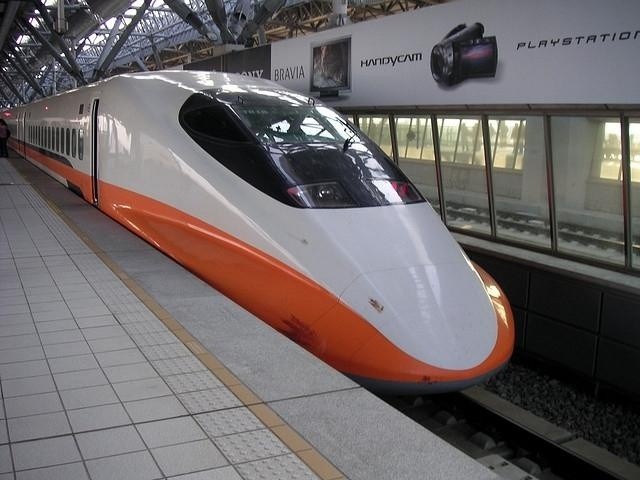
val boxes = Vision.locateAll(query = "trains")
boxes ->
[1,70,516,395]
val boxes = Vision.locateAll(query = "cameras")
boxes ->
[428,21,498,87]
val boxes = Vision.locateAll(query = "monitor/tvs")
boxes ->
[310,34,353,101]
